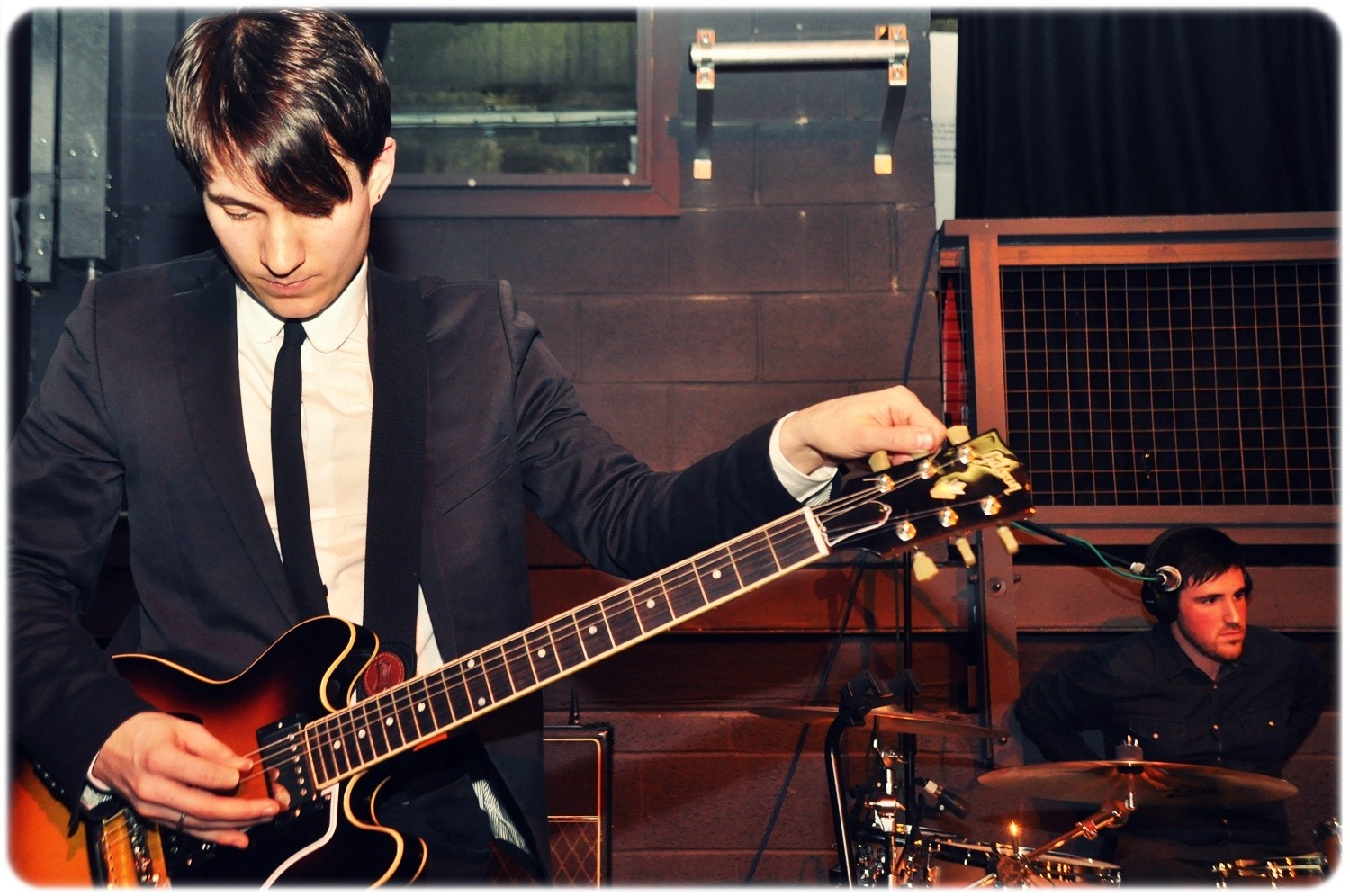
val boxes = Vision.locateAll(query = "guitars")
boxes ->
[9,421,1034,889]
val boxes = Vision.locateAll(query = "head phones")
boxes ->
[1141,524,1253,623]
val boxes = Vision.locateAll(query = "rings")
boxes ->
[175,812,188,831]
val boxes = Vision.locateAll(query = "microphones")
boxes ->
[1154,565,1181,591]
[919,778,971,820]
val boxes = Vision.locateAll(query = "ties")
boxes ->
[270,321,329,623]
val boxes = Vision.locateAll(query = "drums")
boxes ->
[1208,858,1326,890]
[1314,815,1342,881]
[842,822,1124,888]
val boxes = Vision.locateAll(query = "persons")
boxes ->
[1019,528,1325,886]
[8,12,952,888]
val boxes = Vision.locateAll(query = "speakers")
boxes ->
[540,723,614,887]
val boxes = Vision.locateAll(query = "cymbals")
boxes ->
[976,754,1297,812]
[748,702,1010,742]
